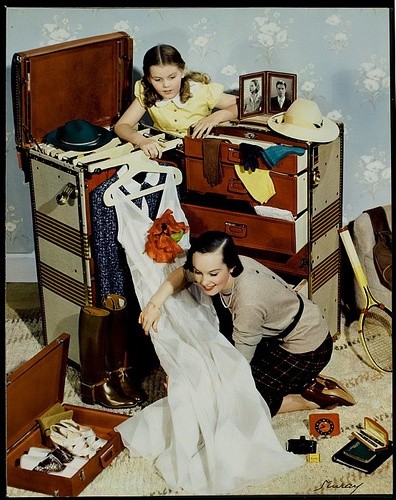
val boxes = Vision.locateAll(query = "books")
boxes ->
[332,437,393,474]
[342,441,376,463]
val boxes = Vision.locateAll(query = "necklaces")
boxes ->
[220,277,234,308]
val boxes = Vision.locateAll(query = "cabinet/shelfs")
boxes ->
[181,112,343,341]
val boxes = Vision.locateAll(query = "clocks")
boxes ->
[309,414,339,436]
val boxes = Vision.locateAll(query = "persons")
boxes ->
[138,231,356,418]
[244,80,262,113]
[114,44,240,160]
[271,80,291,112]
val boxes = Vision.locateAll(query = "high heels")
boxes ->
[60,419,108,450]
[301,377,357,410]
[316,373,354,399]
[49,425,95,459]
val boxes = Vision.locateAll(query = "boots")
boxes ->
[78,306,137,408]
[102,294,148,404]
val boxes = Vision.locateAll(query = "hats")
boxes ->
[267,96,340,144]
[42,118,112,151]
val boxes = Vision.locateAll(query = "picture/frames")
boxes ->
[239,72,265,119]
[266,71,297,115]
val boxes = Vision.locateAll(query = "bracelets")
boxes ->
[148,301,162,314]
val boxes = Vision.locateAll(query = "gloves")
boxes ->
[238,142,263,172]
[202,136,231,186]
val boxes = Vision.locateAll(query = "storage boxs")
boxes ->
[11,30,182,371]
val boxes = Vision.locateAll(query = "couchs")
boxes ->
[353,204,392,312]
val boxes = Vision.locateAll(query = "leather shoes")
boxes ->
[28,446,89,470]
[20,454,78,482]
